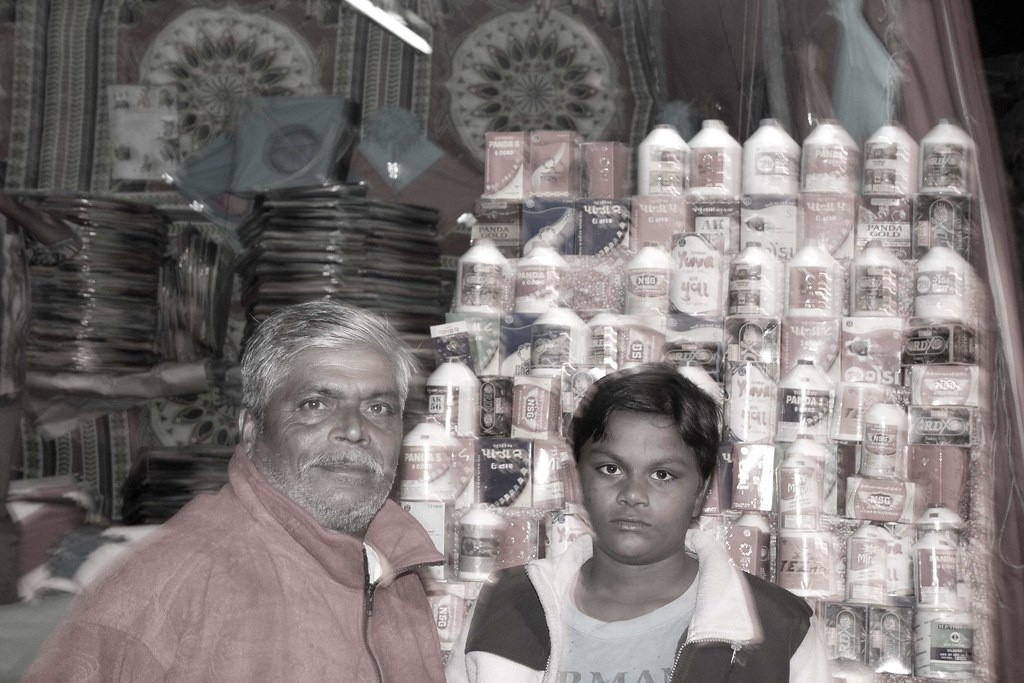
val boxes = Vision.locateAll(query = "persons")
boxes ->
[16,302,452,683]
[445,360,831,683]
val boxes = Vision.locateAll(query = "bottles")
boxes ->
[386,118,978,683]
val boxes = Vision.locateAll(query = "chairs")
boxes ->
[6,476,161,603]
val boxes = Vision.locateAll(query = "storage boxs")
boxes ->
[485,132,530,198]
[573,200,631,253]
[739,194,796,266]
[664,315,725,382]
[798,193,856,268]
[581,142,630,199]
[840,317,903,387]
[446,311,509,376]
[781,318,840,389]
[630,196,694,259]
[520,201,575,255]
[901,324,976,367]
[472,198,522,259]
[426,363,979,676]
[430,321,475,374]
[692,200,740,255]
[854,194,914,266]
[724,315,781,382]
[530,130,583,197]
[912,192,973,262]
